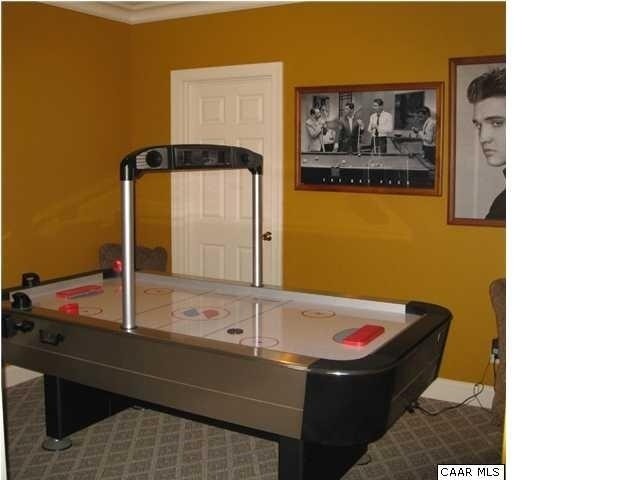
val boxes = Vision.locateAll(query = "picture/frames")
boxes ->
[293,54,506,227]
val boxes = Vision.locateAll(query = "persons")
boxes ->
[305,107,327,152]
[366,99,394,153]
[466,66,506,222]
[411,105,436,164]
[337,102,364,152]
[319,104,336,153]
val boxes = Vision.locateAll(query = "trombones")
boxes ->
[303,158,308,164]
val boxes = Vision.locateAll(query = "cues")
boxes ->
[322,124,325,153]
[357,116,361,153]
[372,118,377,154]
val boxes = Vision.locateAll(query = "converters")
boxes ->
[491,340,500,359]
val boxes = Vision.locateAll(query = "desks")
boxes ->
[1,266,454,479]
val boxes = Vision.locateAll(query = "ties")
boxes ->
[374,113,380,138]
